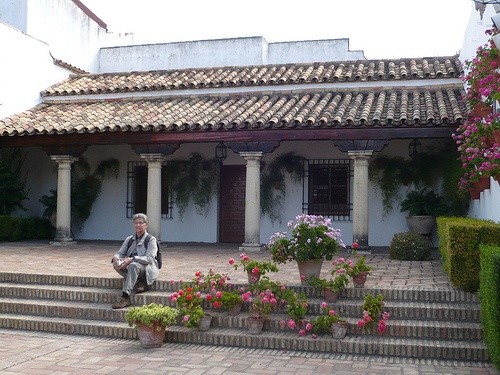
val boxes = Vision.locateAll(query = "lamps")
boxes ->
[215,140,228,165]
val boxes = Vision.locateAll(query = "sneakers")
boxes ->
[113,296,131,308]
[136,282,147,292]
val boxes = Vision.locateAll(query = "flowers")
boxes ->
[451,1,500,193]
[170,212,392,340]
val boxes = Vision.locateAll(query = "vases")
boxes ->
[478,176,490,189]
[491,130,500,142]
[246,271,261,284]
[196,316,213,332]
[351,271,368,289]
[474,181,484,193]
[482,107,492,114]
[246,316,265,335]
[469,188,479,199]
[296,257,324,285]
[322,286,338,304]
[331,321,349,339]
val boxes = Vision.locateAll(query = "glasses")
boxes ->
[135,221,146,225]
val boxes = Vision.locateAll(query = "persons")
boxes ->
[111,213,159,309]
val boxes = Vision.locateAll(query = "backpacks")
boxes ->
[128,233,163,269]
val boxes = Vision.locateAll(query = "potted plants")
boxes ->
[123,302,178,349]
[400,187,443,236]
